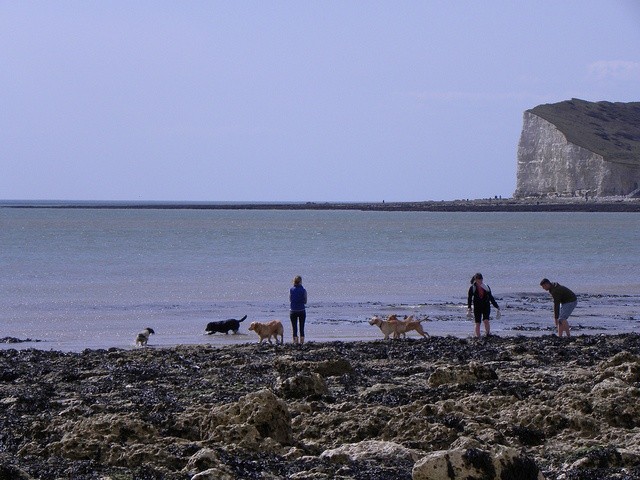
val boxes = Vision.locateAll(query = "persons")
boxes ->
[468,273,500,338]
[289,276,307,344]
[540,278,577,337]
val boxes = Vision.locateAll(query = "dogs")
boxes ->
[387,313,433,340]
[368,313,414,341]
[135,327,155,347]
[247,319,283,347]
[204,313,248,335]
